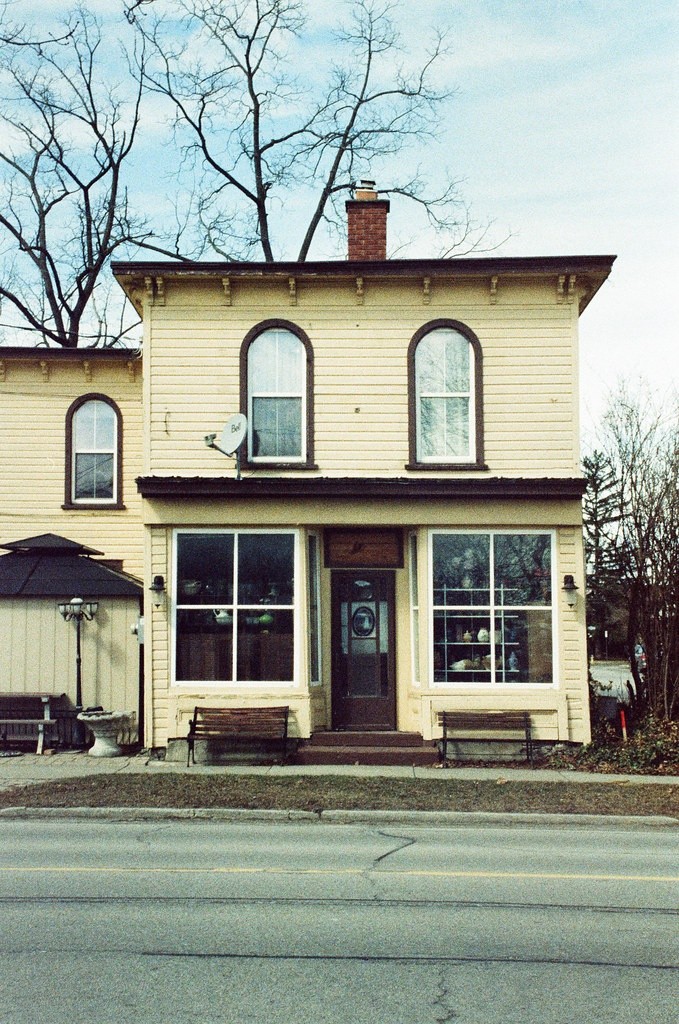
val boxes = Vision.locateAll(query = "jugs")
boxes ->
[213,608,233,626]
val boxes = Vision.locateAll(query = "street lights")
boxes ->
[57,592,99,708]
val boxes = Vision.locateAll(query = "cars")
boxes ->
[629,645,647,672]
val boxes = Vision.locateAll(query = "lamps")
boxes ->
[148,576,166,609]
[561,575,580,608]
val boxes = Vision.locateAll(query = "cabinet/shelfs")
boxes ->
[434,584,522,680]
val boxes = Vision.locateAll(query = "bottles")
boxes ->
[450,627,518,670]
[259,611,273,623]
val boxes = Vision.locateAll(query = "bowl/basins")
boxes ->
[177,579,202,596]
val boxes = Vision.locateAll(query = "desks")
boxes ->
[0,691,66,754]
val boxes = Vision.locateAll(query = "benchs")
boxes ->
[438,711,536,767]
[168,705,290,767]
[0,719,57,725]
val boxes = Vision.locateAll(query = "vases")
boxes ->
[77,711,130,757]
